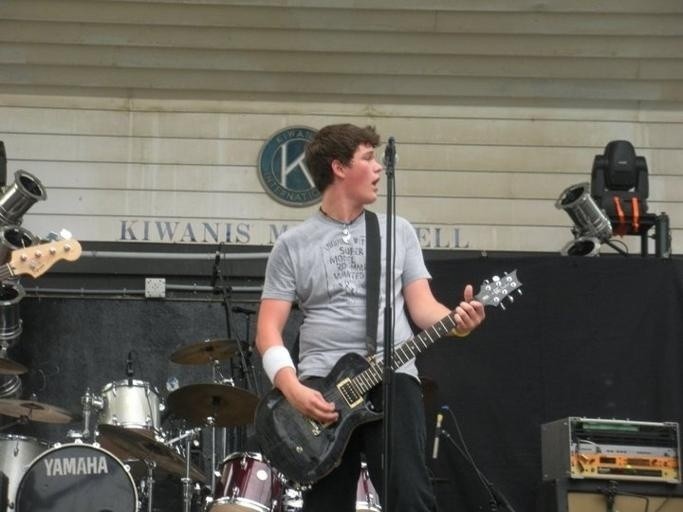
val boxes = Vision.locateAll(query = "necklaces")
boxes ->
[318,205,367,244]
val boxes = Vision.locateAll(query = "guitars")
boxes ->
[253,269,522,484]
[0,230,82,281]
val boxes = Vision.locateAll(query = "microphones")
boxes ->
[231,306,256,315]
[381,136,399,168]
[210,245,221,286]
[126,352,133,386]
[432,408,444,460]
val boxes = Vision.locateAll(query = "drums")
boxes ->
[202,451,286,512]
[16,438,154,512]
[0,432,53,511]
[96,379,161,462]
[286,458,383,511]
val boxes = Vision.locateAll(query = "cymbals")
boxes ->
[164,385,260,427]
[98,425,213,484]
[0,398,83,424]
[169,338,254,365]
[0,356,29,375]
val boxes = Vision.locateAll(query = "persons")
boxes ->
[255,124,487,512]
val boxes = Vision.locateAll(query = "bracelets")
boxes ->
[261,344,298,387]
[451,327,474,338]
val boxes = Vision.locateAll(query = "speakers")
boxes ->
[535,478,683,512]
